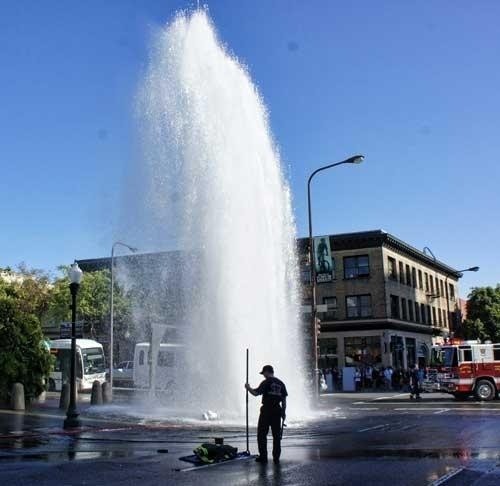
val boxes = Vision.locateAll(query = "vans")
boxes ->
[133,343,186,401]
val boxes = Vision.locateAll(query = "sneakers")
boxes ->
[410,395,421,400]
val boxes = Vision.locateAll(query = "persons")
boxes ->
[245,365,288,464]
[319,361,428,400]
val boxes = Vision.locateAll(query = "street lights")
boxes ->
[67,263,81,431]
[307,154,365,405]
[108,241,139,400]
[445,265,480,336]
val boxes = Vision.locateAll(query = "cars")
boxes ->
[110,361,133,384]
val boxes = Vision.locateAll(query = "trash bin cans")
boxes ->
[326,373,336,393]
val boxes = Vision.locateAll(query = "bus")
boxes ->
[47,339,106,391]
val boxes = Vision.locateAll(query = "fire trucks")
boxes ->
[426,342,500,401]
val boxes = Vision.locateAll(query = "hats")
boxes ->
[259,365,274,374]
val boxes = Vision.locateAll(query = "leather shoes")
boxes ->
[256,456,281,464]
